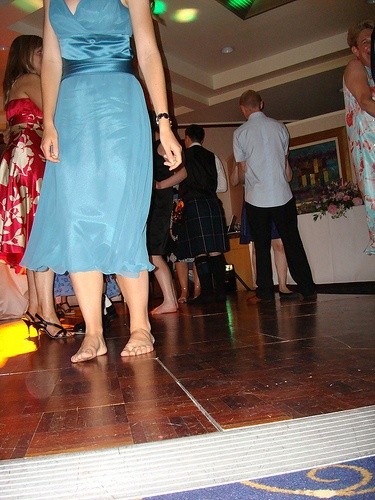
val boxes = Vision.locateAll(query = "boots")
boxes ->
[192,256,214,304]
[210,254,228,302]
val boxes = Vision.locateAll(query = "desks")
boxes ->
[249,205,375,288]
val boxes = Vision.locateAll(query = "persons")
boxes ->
[19,0,183,363]
[0,34,77,341]
[51,269,123,335]
[175,124,228,304]
[341,18,375,258]
[233,89,318,306]
[164,172,201,303]
[229,151,297,297]
[144,110,188,315]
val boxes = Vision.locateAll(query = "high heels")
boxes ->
[58,302,75,316]
[32,313,74,340]
[22,311,38,332]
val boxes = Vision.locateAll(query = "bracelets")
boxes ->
[155,113,173,127]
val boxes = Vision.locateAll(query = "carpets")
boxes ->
[1,405,375,500]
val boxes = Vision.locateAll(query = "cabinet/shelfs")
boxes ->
[223,233,253,291]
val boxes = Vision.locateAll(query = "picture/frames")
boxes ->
[227,125,353,225]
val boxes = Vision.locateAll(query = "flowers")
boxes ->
[308,178,363,221]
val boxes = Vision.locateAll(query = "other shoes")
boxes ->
[246,295,271,302]
[279,290,301,297]
[72,314,111,335]
[177,297,188,304]
